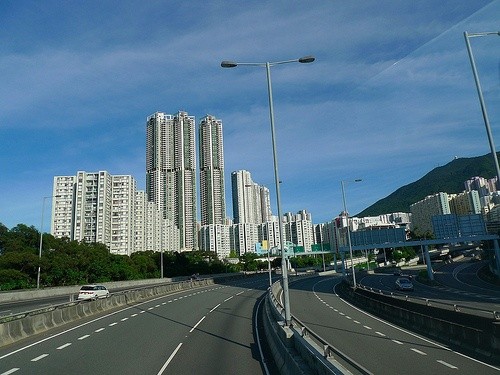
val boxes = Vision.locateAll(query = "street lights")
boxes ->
[160,225,171,279]
[37,195,61,289]
[245,181,283,287]
[221,55,316,325]
[341,179,363,289]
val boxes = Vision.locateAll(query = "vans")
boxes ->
[77,284,109,300]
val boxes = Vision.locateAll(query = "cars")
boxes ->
[394,277,413,291]
[391,265,421,277]
